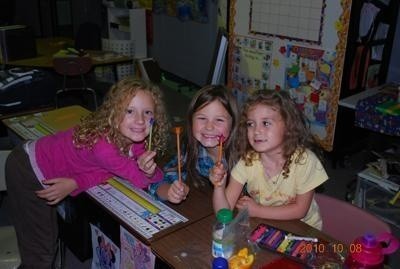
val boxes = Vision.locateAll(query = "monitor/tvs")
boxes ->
[202,26,231,87]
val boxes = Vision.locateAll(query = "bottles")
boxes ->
[212,208,236,259]
[349,231,400,269]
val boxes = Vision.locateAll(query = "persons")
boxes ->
[147,83,240,205]
[210,89,329,231]
[4,77,170,269]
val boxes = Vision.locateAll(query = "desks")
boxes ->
[0,103,215,246]
[149,207,349,269]
[8,50,132,82]
[338,82,400,200]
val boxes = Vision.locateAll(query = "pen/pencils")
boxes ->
[218,134,224,166]
[148,118,153,151]
[176,128,182,185]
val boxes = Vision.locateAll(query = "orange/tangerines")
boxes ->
[227,247,255,269]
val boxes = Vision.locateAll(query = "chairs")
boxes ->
[313,191,391,249]
[52,55,97,111]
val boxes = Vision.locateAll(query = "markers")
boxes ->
[252,226,318,260]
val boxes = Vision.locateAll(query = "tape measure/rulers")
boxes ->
[41,124,190,223]
[31,124,161,217]
